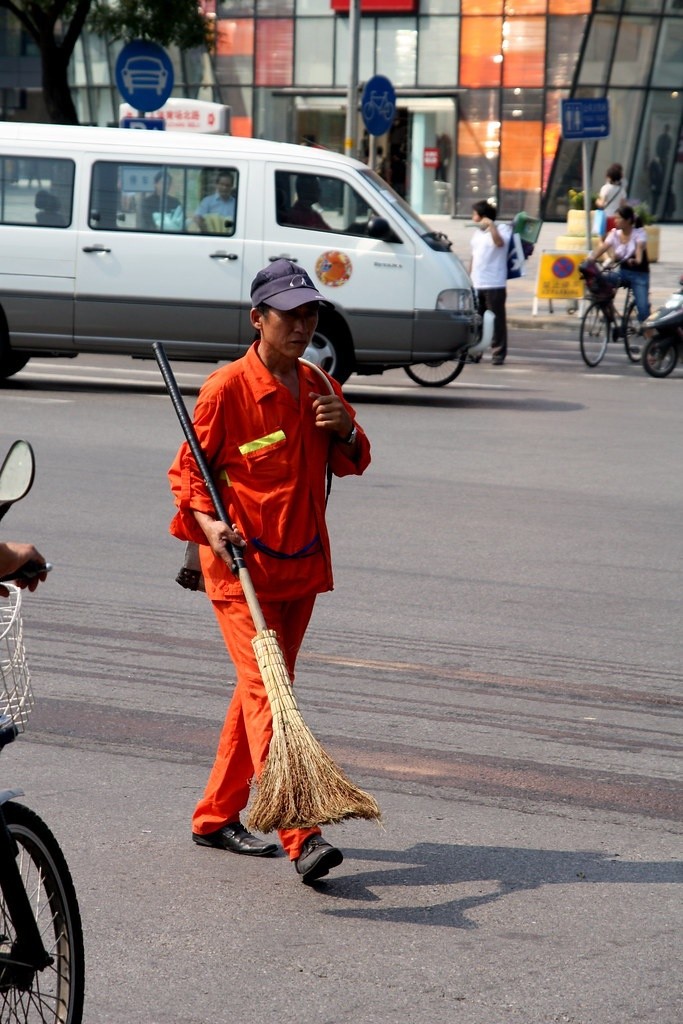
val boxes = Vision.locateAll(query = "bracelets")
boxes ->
[335,423,357,444]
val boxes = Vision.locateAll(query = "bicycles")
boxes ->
[575,256,654,367]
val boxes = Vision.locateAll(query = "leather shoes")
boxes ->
[192,821,278,856]
[294,834,344,883]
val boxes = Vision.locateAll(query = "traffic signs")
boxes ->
[560,97,610,139]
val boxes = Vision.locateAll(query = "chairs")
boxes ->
[277,190,291,224]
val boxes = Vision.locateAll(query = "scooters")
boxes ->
[0,435,91,1024]
[636,276,683,379]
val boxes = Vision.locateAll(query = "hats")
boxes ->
[474,201,496,223]
[606,163,622,182]
[250,258,335,311]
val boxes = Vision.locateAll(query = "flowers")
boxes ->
[626,199,657,227]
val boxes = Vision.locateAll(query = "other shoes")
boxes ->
[600,307,618,322]
[464,354,482,363]
[491,357,504,365]
[647,355,658,365]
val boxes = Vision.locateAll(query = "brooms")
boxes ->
[150,341,383,835]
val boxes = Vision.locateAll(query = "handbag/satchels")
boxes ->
[507,233,525,279]
[175,540,206,595]
[591,209,606,236]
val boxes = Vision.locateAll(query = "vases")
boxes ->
[636,226,660,260]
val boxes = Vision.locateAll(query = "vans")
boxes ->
[0,118,483,391]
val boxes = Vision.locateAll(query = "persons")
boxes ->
[649,157,662,214]
[284,174,363,233]
[589,207,656,364]
[364,147,405,198]
[436,131,450,182]
[193,172,235,234]
[594,163,628,266]
[656,124,672,164]
[141,172,180,230]
[301,135,314,147]
[169,259,372,883]
[468,200,512,365]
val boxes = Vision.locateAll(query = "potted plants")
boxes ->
[567,189,603,239]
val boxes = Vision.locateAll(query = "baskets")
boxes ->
[583,270,622,303]
[0,581,35,736]
